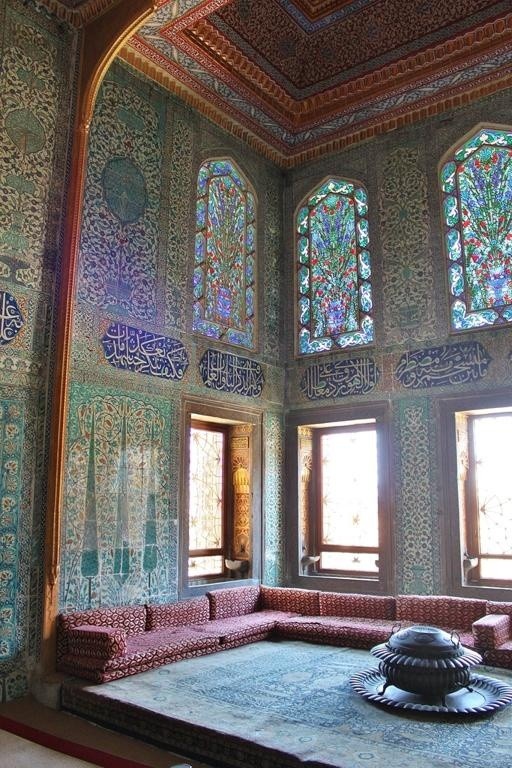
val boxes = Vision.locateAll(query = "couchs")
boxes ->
[56,585,512,684]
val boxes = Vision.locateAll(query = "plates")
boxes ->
[350,668,512,717]
